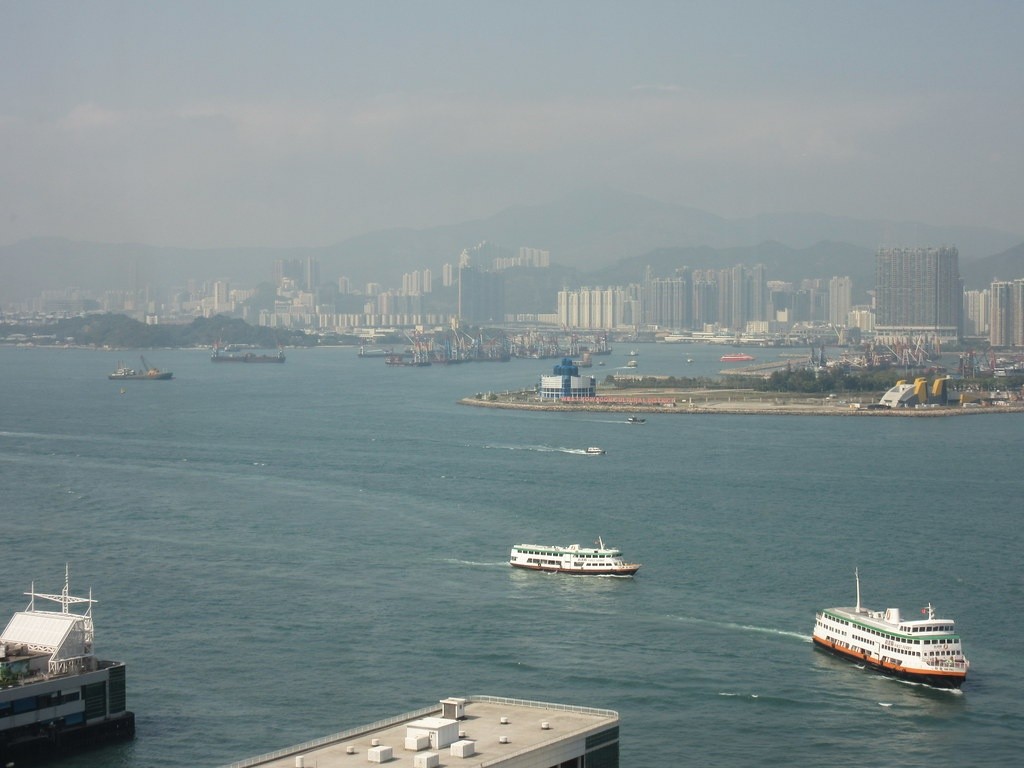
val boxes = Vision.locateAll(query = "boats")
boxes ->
[361,324,614,367]
[720,352,754,362]
[584,446,606,454]
[627,413,648,425]
[810,565,970,688]
[210,336,288,364]
[508,532,642,577]
[627,360,637,368]
[107,354,174,380]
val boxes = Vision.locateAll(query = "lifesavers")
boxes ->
[880,660,884,665]
[538,563,541,567]
[902,669,906,673]
